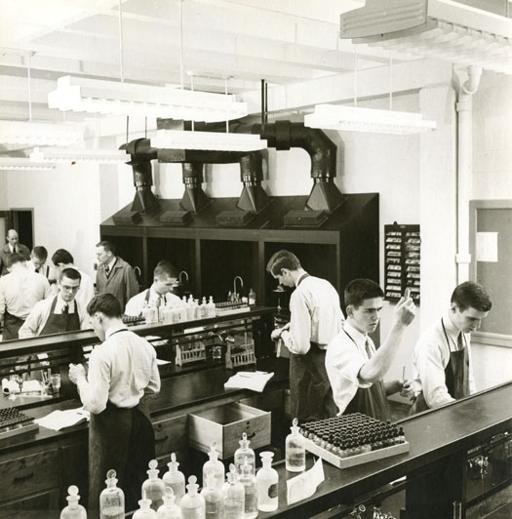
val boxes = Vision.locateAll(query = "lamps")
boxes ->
[338,1,512,73]
[0,64,66,175]
[302,72,428,137]
[149,75,269,152]
[54,1,236,123]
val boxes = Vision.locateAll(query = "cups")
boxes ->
[211,346,222,360]
[50,373,62,389]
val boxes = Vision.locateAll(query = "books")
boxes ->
[1,378,41,396]
[32,405,90,432]
[223,368,275,394]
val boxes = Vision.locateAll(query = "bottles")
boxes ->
[299,411,406,456]
[57,435,280,519]
[285,417,306,472]
[141,293,218,325]
[248,289,257,306]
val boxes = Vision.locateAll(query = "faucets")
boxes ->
[133,265,142,277]
[233,274,244,295]
[179,270,189,283]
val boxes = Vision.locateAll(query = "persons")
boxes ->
[323,278,418,425]
[266,248,346,421]
[67,291,162,517]
[51,248,95,330]
[410,280,494,414]
[0,252,51,340]
[124,256,187,316]
[27,245,54,281]
[94,240,141,315]
[0,229,31,275]
[17,267,94,338]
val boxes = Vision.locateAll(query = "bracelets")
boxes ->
[279,329,287,338]
